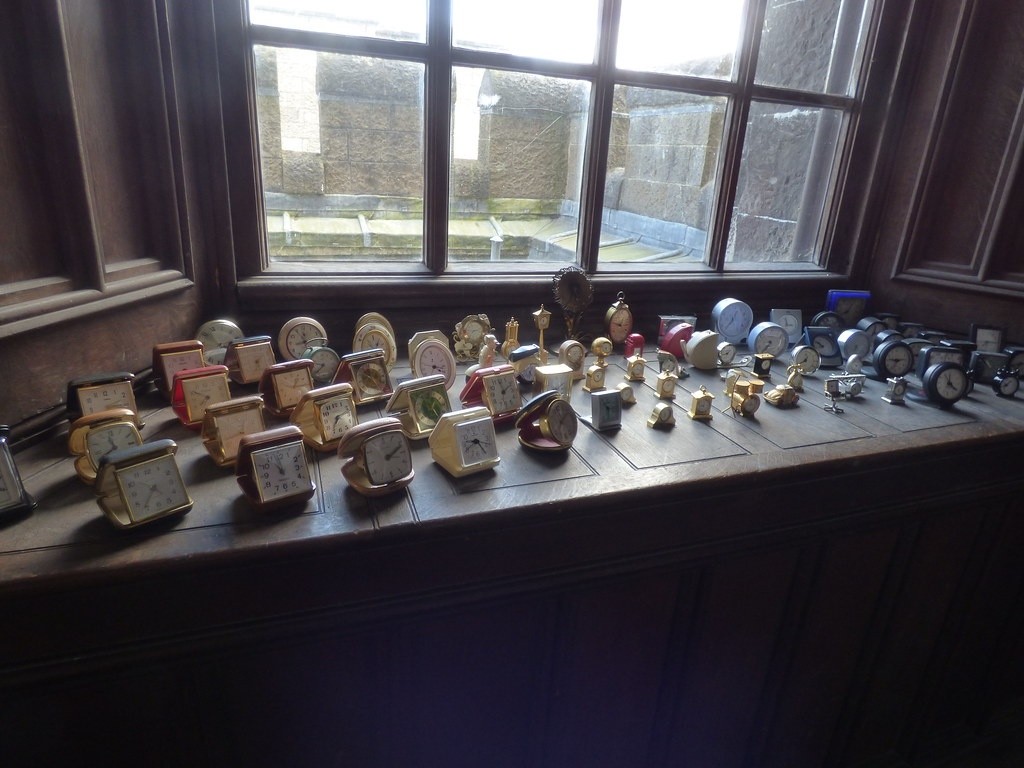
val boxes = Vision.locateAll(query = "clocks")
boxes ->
[66,370,146,431]
[152,341,207,402]
[301,336,339,387]
[234,425,318,513]
[409,330,456,391]
[0,425,38,530]
[353,313,397,372]
[203,391,267,469]
[195,317,244,366]
[223,335,277,390]
[337,413,415,499]
[171,365,232,430]
[427,267,1024,478]
[68,408,143,486]
[94,439,194,534]
[386,374,452,441]
[278,316,329,362]
[289,382,357,452]
[329,348,393,409]
[258,358,315,420]
